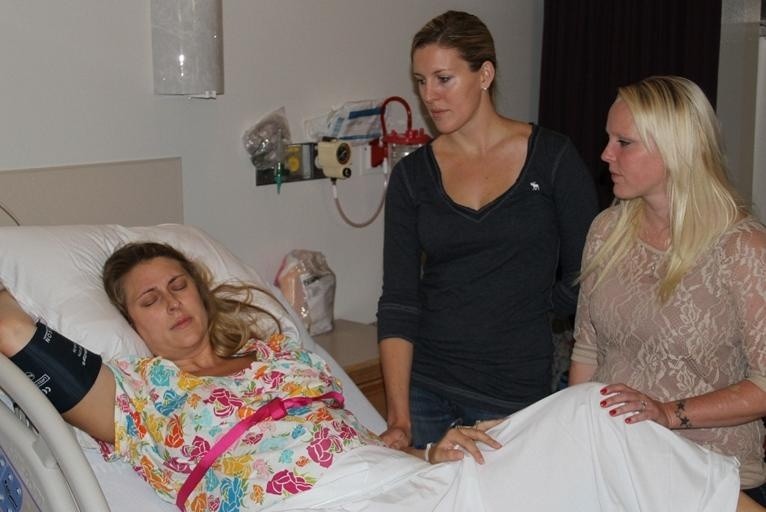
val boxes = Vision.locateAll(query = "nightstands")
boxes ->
[310,318,389,441]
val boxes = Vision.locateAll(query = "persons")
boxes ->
[376,9,601,450]
[0,241,766,512]
[567,75,766,510]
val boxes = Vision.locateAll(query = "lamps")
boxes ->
[150,0,225,97]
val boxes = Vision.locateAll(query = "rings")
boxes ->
[475,419,481,425]
[638,397,647,408]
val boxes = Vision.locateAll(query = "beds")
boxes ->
[1,222,387,510]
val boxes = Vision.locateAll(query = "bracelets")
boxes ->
[423,436,433,461]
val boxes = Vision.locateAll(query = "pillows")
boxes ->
[1,223,303,450]
